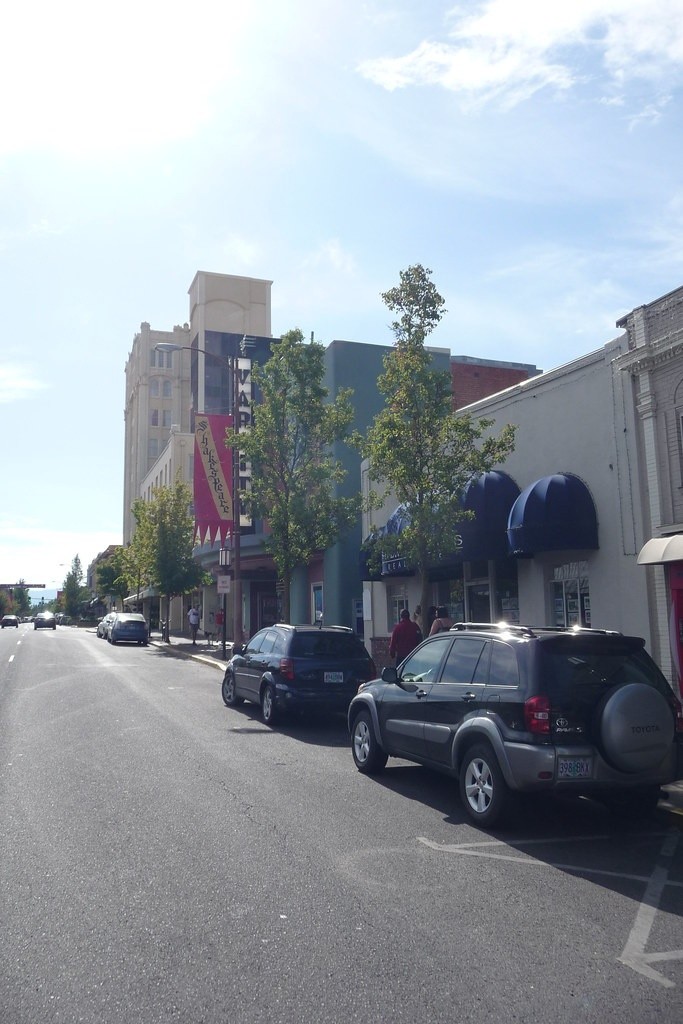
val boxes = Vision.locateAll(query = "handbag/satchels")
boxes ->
[416,623,423,644]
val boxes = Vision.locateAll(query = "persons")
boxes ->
[187,604,201,645]
[389,604,452,668]
[215,608,224,643]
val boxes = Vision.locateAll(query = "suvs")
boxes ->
[95,612,149,646]
[220,621,377,726]
[346,621,683,831]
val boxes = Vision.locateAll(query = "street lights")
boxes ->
[218,546,233,659]
[154,342,243,655]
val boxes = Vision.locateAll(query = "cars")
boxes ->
[1,614,20,628]
[22,611,88,627]
[33,612,57,630]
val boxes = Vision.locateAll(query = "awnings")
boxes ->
[382,470,521,577]
[358,532,388,582]
[507,474,599,551]
[636,533,683,565]
